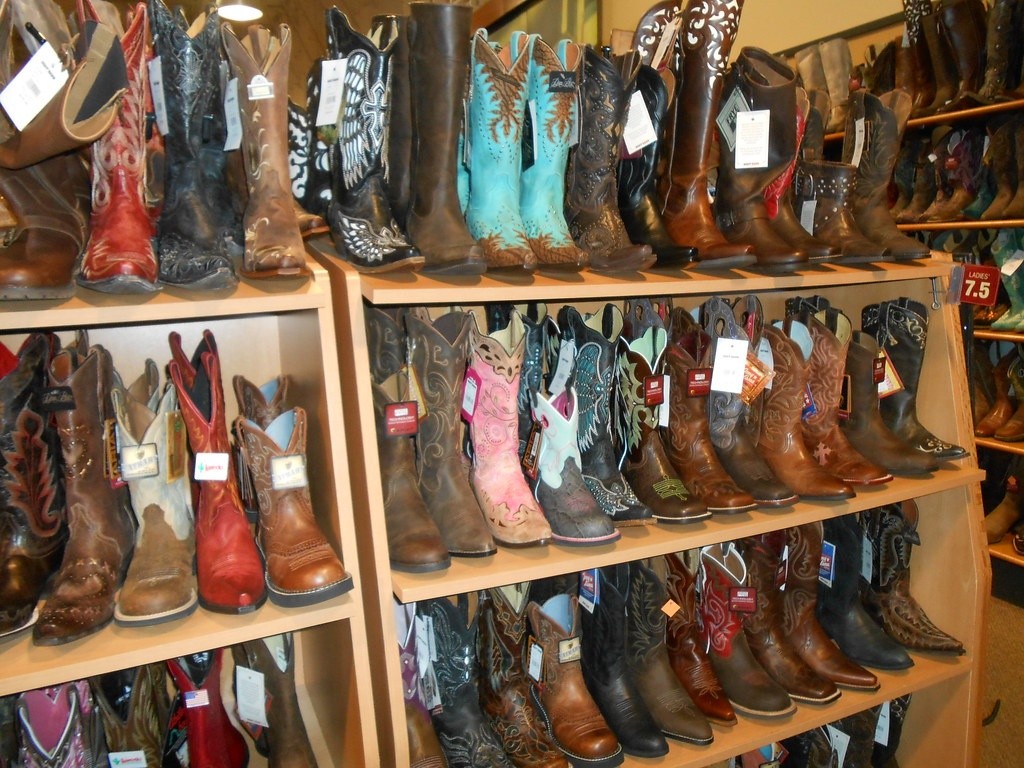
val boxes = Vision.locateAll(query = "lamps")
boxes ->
[219,0,264,22]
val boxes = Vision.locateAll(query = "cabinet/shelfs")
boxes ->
[823,99,1024,567]
[0,261,378,768]
[300,237,992,768]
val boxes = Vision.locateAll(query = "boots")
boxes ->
[0,0,1024,768]
